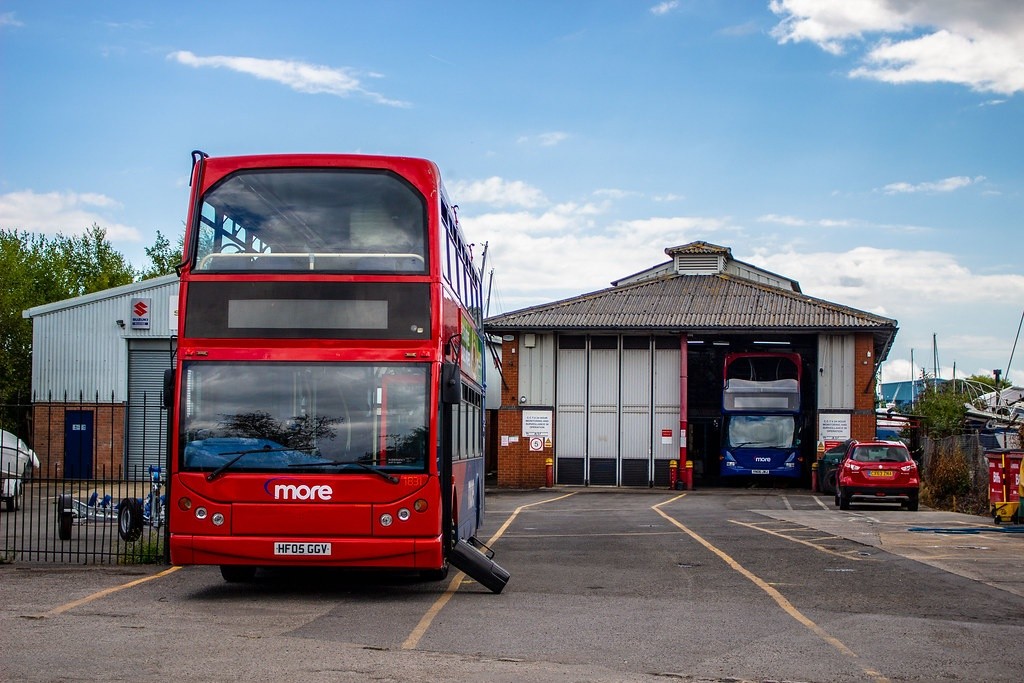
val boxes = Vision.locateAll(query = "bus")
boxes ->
[722,351,802,484]
[168,150,510,593]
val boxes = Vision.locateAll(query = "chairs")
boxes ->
[855,448,870,462]
[886,448,900,461]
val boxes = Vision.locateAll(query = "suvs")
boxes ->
[818,442,849,494]
[836,438,919,512]
